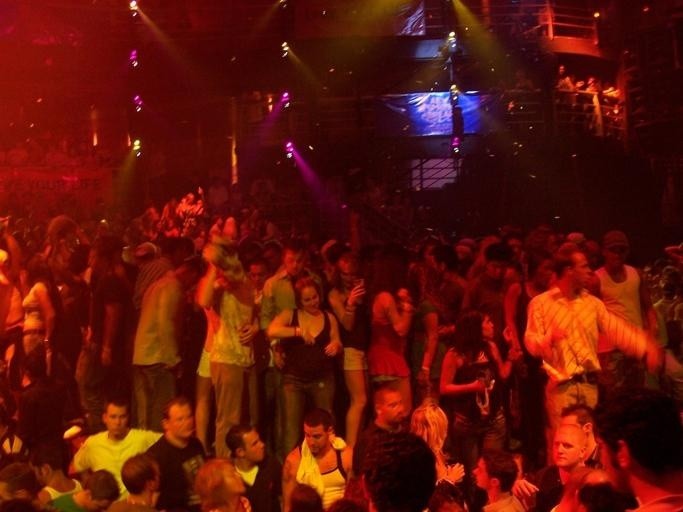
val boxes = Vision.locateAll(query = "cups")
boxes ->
[354,279,364,305]
[451,463,463,483]
[521,492,537,510]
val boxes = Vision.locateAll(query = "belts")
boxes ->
[573,374,598,383]
[21,330,43,336]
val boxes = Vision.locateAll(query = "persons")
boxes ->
[515,64,623,132]
[1,151,682,510]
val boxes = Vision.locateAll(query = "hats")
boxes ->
[604,230,628,250]
[326,242,351,265]
